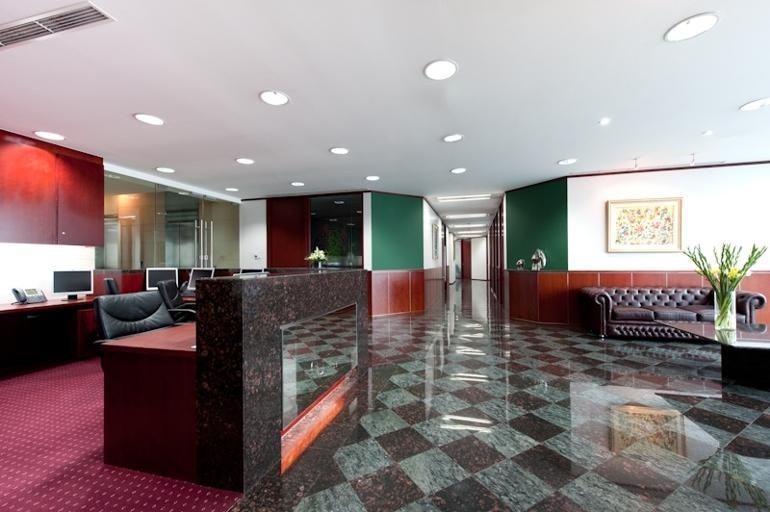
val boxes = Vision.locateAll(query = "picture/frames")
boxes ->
[602,198,683,255]
[600,400,692,465]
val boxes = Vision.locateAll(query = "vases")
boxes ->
[709,289,739,332]
[311,258,324,269]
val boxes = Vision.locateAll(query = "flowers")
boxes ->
[303,245,328,262]
[680,239,768,325]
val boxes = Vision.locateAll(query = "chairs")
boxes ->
[90,271,198,375]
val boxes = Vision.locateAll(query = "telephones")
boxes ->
[12,288,48,304]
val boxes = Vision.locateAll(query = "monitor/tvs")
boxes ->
[52,269,94,301]
[145,268,179,291]
[187,268,215,291]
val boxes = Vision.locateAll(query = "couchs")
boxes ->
[573,282,766,347]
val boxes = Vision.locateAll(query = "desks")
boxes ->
[89,289,197,308]
[0,294,97,369]
[99,315,200,485]
